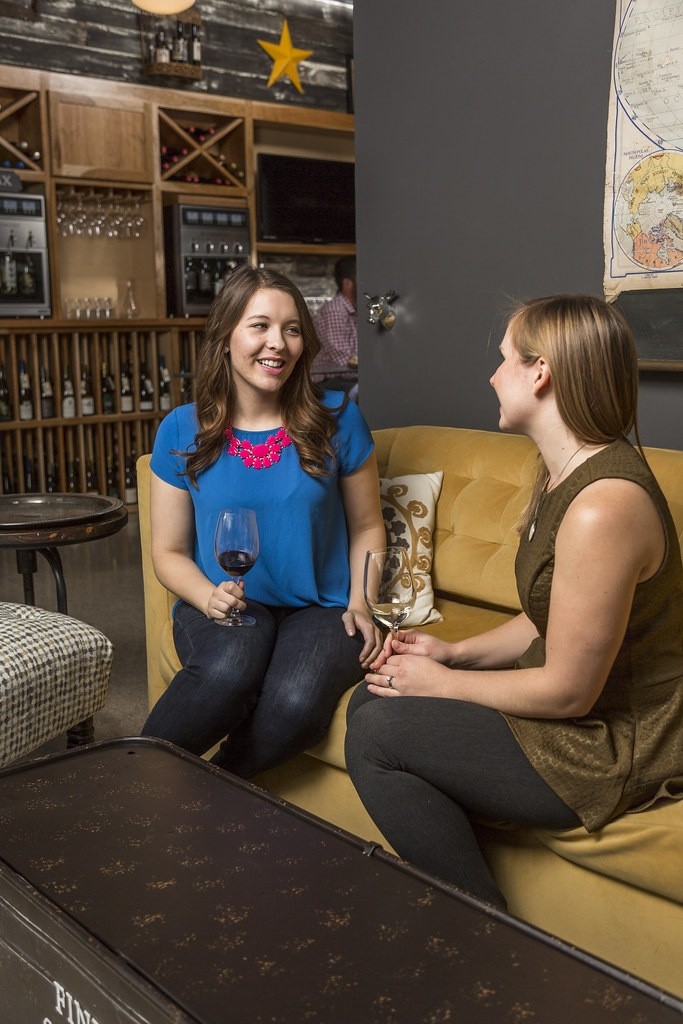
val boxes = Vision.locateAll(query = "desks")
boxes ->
[0,493,128,616]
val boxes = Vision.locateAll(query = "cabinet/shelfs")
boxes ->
[0,317,211,514]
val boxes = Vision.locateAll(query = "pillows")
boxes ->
[376,470,445,629]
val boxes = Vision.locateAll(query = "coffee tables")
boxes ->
[0,735,683,1024]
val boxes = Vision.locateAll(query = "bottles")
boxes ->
[120,277,139,319]
[0,252,35,301]
[0,138,40,168]
[185,256,245,302]
[154,21,203,66]
[1,356,189,505]
[160,125,245,185]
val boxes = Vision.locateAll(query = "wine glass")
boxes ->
[363,546,416,674]
[213,509,260,627]
[55,190,146,239]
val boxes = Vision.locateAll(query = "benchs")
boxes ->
[0,601,115,769]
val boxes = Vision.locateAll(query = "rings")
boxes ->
[387,675,393,688]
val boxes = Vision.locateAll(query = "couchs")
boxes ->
[135,426,683,1000]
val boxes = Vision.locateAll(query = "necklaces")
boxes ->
[526,440,593,540]
[223,413,291,469]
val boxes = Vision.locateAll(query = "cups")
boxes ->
[61,298,113,319]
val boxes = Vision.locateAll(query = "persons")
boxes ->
[307,255,358,402]
[341,289,683,910]
[140,266,389,784]
